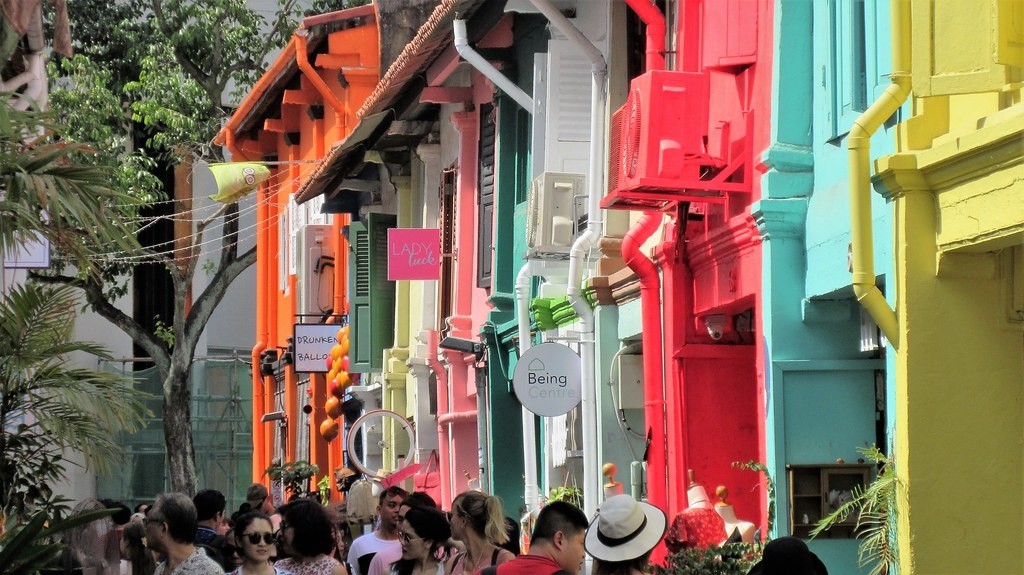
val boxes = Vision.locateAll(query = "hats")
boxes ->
[371,469,392,483]
[584,494,667,561]
[746,537,828,575]
[131,513,146,521]
[246,483,268,508]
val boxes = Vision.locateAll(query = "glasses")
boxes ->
[241,531,275,545]
[396,531,426,547]
[446,511,461,524]
[279,521,294,533]
[142,517,164,528]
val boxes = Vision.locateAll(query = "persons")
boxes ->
[97,482,352,575]
[583,494,666,575]
[346,485,409,575]
[368,491,448,575]
[665,468,728,553]
[713,486,755,566]
[747,537,829,575]
[273,500,348,575]
[473,501,589,575]
[445,490,515,575]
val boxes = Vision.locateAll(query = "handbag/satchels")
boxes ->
[414,448,442,511]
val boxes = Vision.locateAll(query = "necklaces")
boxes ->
[464,544,484,572]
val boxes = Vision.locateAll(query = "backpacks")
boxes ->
[346,476,385,536]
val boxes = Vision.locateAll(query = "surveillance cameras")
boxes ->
[707,325,723,340]
